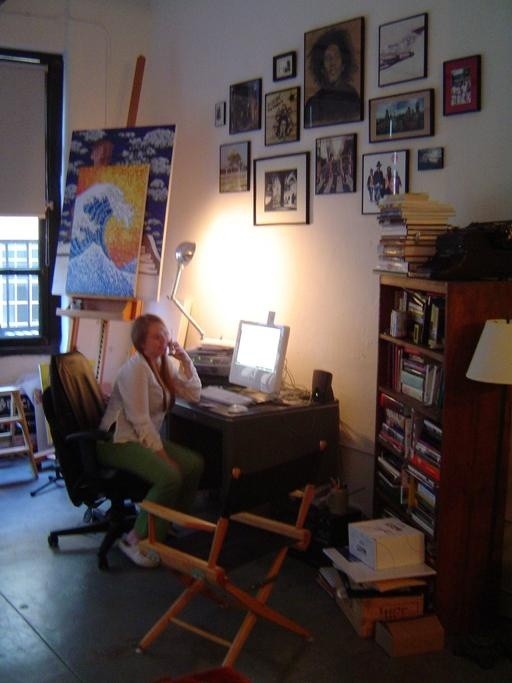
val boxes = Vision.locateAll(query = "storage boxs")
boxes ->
[376,612,445,658]
[336,569,423,639]
[348,518,425,569]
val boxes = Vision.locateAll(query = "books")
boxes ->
[196,337,234,357]
[374,456,436,538]
[373,193,459,279]
[387,289,445,351]
[375,400,440,487]
[381,341,443,412]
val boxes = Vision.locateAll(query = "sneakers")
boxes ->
[121,536,158,568]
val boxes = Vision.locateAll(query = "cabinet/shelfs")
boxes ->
[371,269,511,608]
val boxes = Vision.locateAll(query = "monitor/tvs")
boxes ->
[229,320,290,394]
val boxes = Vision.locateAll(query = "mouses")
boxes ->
[227,404,248,413]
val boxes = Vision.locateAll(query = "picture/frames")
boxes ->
[443,53,482,116]
[219,140,250,193]
[315,134,357,196]
[229,77,261,135]
[265,86,300,146]
[273,51,297,83]
[50,124,177,302]
[65,163,150,301]
[378,11,427,88]
[254,150,309,228]
[215,101,226,127]
[368,88,435,143]
[301,15,364,129]
[361,148,411,215]
[417,145,444,172]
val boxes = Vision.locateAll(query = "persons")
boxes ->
[94,314,205,569]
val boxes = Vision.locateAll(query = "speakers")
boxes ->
[312,370,334,403]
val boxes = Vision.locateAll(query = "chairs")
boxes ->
[41,350,155,570]
[135,484,314,671]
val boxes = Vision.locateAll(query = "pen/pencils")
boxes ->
[329,475,348,491]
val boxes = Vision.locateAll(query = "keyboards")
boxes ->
[201,385,252,407]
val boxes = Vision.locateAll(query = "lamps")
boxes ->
[452,318,512,671]
[167,241,204,344]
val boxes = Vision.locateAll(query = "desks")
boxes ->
[170,382,340,508]
